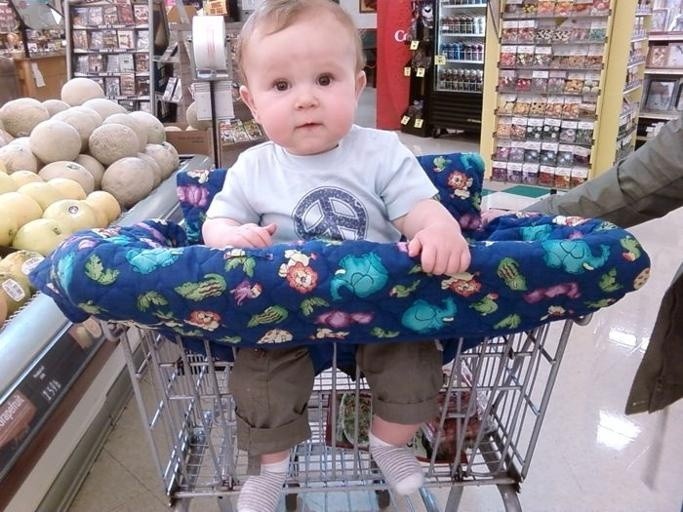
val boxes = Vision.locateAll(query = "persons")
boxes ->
[203,0,471,512]
[520,81,683,511]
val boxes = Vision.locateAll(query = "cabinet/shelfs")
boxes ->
[636,0,683,153]
[481,0,653,190]
[0,152,216,511]
[64,0,175,122]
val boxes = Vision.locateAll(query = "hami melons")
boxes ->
[0,159,122,259]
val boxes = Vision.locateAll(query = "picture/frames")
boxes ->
[359,0,377,14]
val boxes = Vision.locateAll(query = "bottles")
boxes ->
[441,40,483,61]
[439,69,483,92]
[442,15,485,33]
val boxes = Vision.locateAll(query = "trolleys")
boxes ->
[52,147,643,504]
